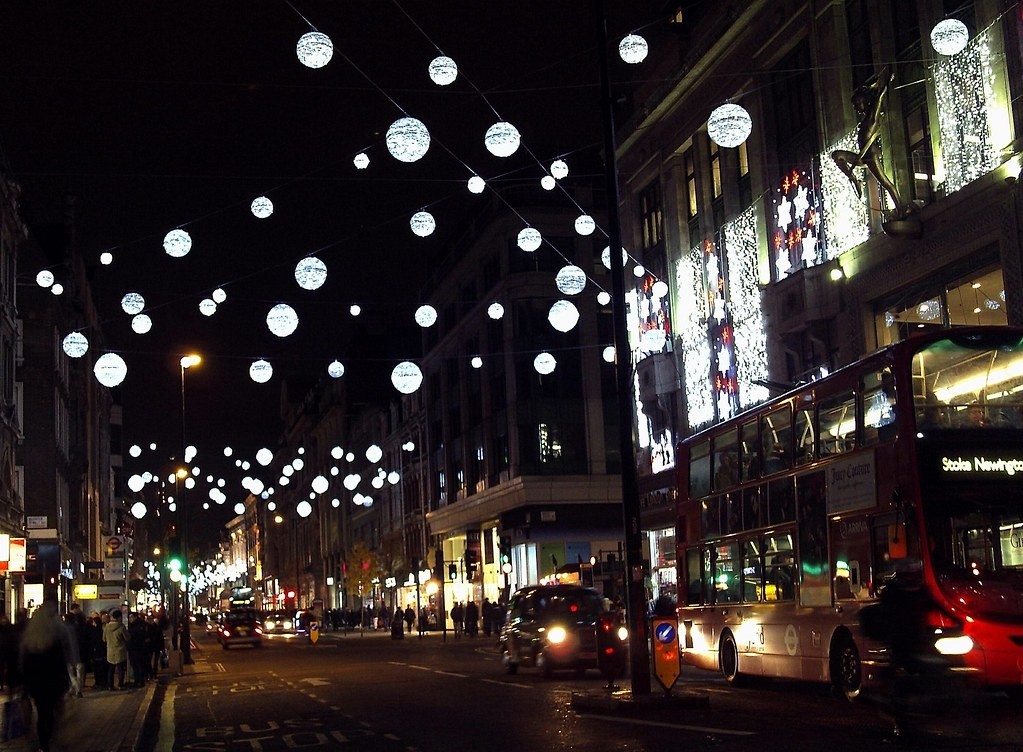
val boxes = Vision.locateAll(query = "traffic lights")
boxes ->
[464,548,479,574]
[500,535,513,575]
[448,564,458,580]
[167,537,182,570]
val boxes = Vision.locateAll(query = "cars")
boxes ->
[498,584,623,682]
[204,611,223,635]
[214,607,264,648]
[262,614,294,634]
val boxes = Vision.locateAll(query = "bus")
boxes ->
[671,326,1023,705]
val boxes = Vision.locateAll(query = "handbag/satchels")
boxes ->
[1,687,31,742]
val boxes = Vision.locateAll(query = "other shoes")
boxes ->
[118,686,126,690]
[109,687,113,691]
[77,691,83,698]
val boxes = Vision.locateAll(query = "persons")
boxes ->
[654,583,675,615]
[0,601,165,752]
[717,452,753,488]
[918,406,942,433]
[960,405,984,429]
[301,598,508,646]
